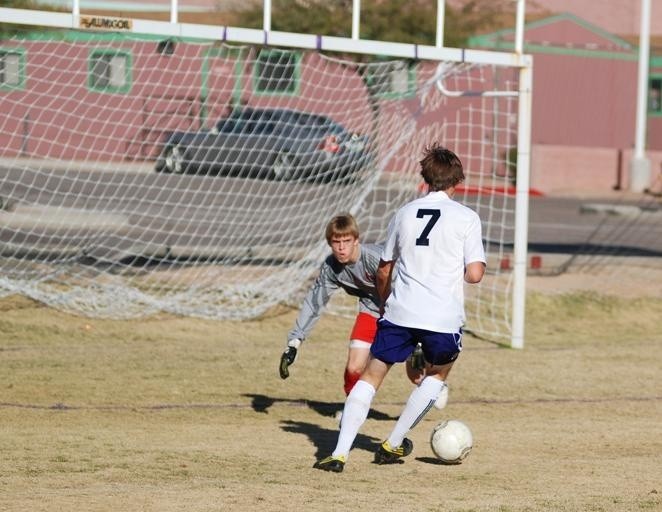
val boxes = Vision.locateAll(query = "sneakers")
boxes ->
[434,380,451,411]
[373,437,416,465]
[317,452,345,474]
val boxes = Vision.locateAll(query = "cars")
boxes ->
[152,111,367,182]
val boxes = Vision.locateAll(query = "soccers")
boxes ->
[431,419,472,464]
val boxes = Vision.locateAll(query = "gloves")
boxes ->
[275,339,301,380]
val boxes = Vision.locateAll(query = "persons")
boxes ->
[314,141,487,473]
[278,212,451,430]
